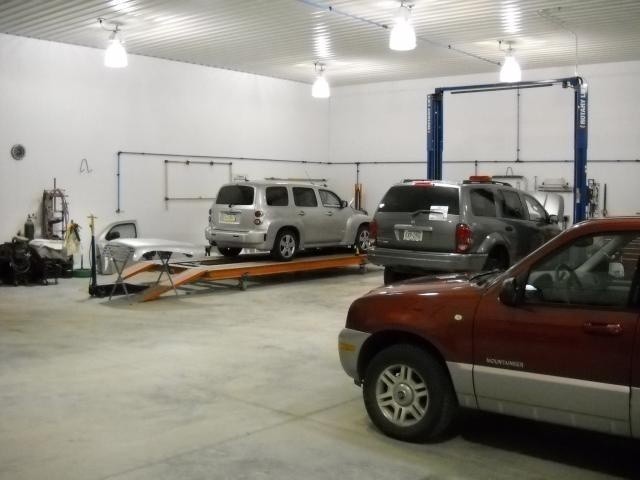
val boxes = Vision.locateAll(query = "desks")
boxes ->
[101,238,194,305]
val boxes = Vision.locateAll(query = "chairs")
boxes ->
[625,256,640,313]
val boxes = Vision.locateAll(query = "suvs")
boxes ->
[205,176,373,259]
[337,215,640,459]
[365,177,564,287]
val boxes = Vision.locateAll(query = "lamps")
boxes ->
[388,0,418,53]
[311,60,331,97]
[96,15,130,70]
[497,38,523,84]
